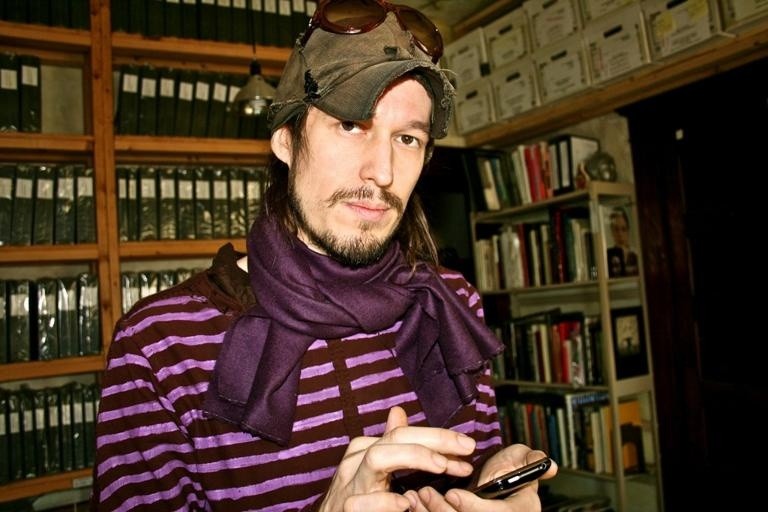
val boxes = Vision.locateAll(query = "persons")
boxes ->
[91,0,563,511]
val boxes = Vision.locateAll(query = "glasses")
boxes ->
[299,0,444,65]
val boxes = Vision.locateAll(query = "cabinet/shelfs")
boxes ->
[103,0,318,360]
[468,113,666,512]
[0,2,115,512]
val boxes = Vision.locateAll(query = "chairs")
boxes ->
[31,486,94,512]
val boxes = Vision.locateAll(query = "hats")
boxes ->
[265,11,457,140]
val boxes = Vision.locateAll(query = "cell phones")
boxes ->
[471,458,551,499]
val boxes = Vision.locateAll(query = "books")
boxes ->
[477,136,601,211]
[477,196,640,294]
[496,389,646,475]
[490,308,648,387]
